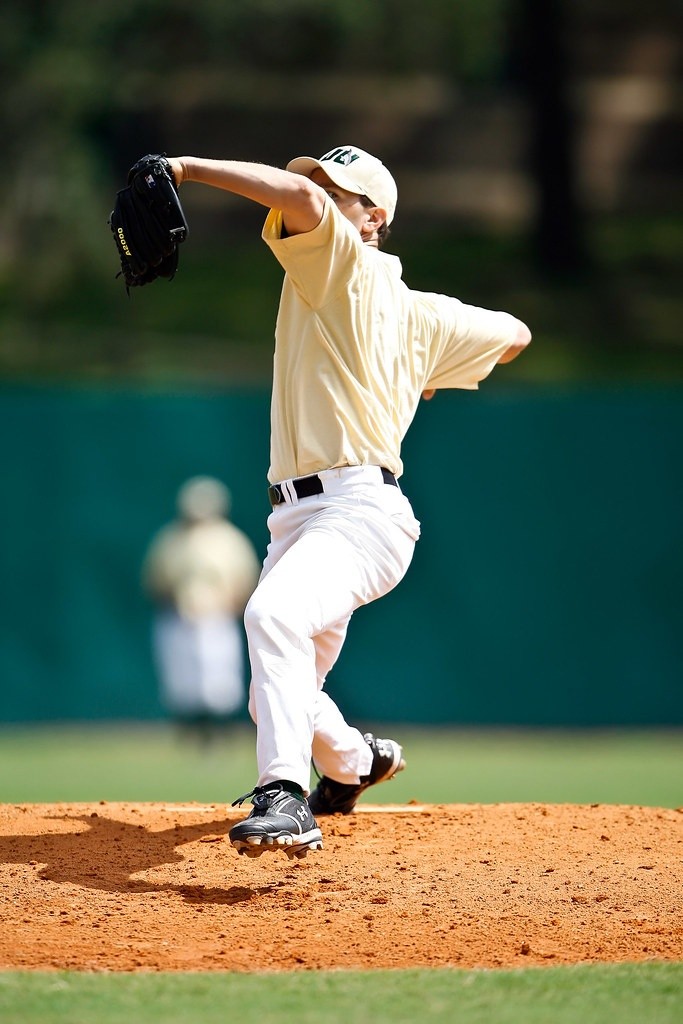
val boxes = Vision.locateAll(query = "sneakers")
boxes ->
[306,733,406,815]
[229,783,323,860]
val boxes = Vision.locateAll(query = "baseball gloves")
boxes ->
[109,154,190,289]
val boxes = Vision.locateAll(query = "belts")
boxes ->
[268,467,398,506]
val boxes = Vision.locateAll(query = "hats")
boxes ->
[286,145,397,226]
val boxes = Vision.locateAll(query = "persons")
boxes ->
[110,144,532,858]
[137,475,263,754]
